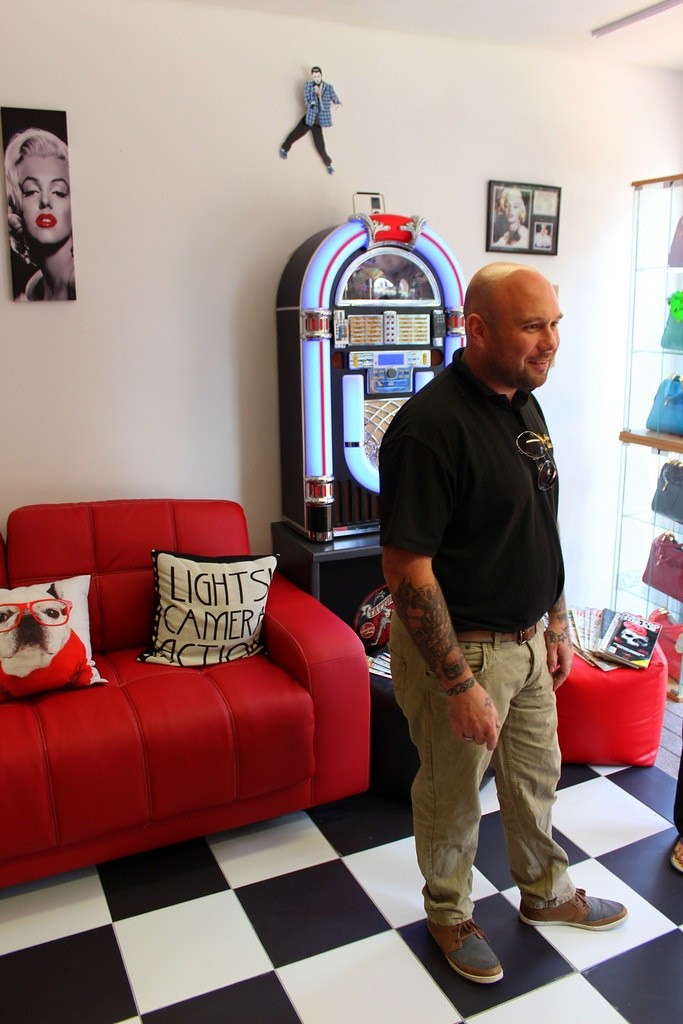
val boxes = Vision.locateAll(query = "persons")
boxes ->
[670,733,683,872]
[5,129,76,302]
[494,189,528,246]
[535,225,551,248]
[378,263,628,984]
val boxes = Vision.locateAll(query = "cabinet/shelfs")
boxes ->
[619,430,683,702]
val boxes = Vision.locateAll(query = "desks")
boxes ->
[271,521,382,601]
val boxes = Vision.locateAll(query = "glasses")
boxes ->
[516,430,558,491]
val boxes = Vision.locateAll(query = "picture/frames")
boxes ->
[485,180,562,256]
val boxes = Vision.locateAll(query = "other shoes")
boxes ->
[670,837,683,875]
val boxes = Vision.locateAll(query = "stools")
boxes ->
[554,641,669,767]
[370,644,495,802]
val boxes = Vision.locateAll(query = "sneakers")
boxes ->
[519,887,628,931]
[427,919,503,984]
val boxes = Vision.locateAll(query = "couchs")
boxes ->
[0,498,371,890]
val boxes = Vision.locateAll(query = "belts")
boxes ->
[457,624,536,646]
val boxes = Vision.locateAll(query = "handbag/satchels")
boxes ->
[668,216,683,267]
[646,373,683,436]
[661,291,683,350]
[642,531,683,603]
[647,608,683,683]
[651,459,683,525]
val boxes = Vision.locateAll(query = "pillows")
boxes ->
[1,575,108,702]
[137,548,280,667]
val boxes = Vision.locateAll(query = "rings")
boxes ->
[464,737,473,740]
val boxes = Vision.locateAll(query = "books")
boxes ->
[367,653,391,679]
[543,608,662,671]
